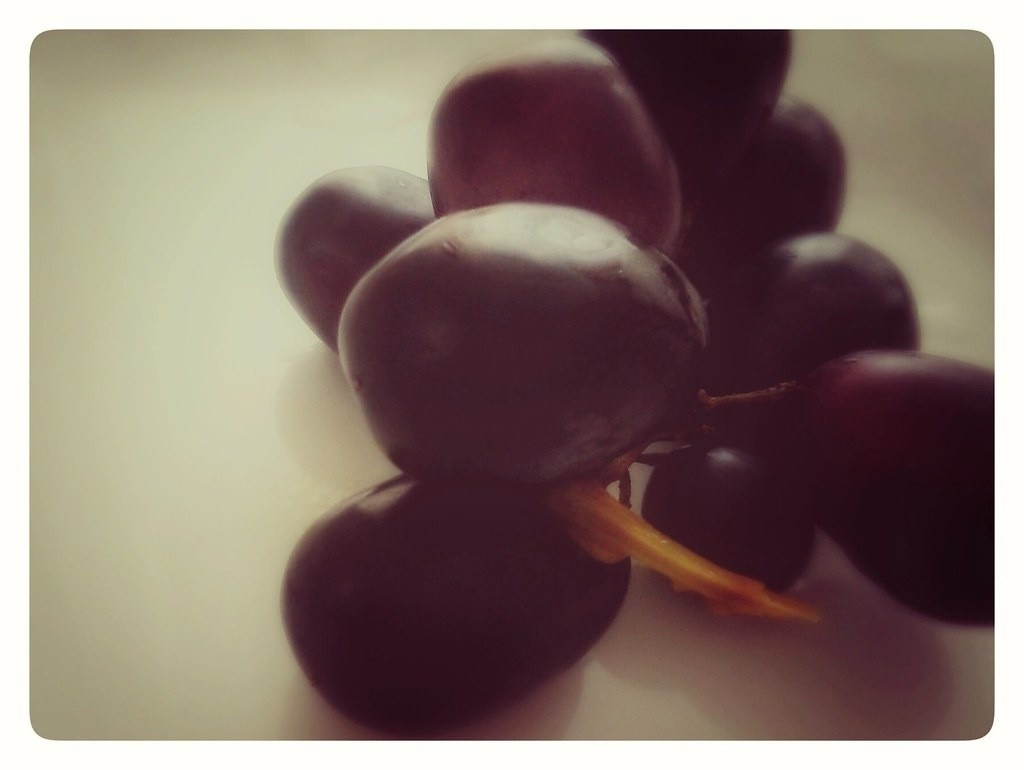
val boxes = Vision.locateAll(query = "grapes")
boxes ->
[280,30,994,739]
